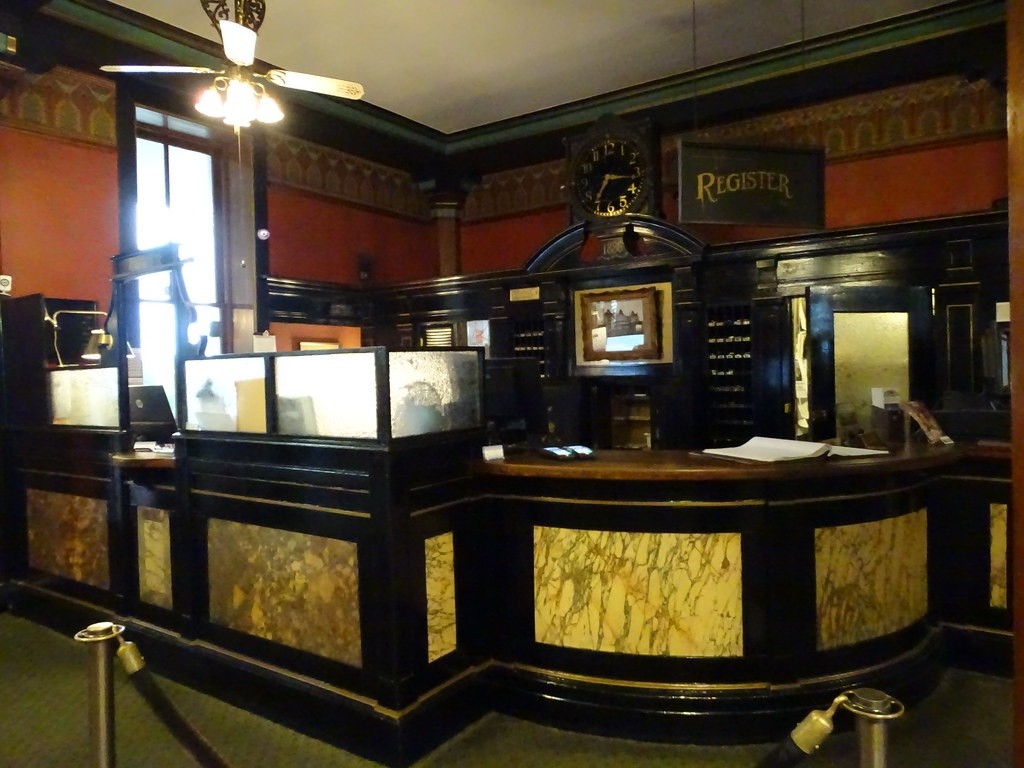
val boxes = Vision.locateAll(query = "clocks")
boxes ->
[562,110,663,229]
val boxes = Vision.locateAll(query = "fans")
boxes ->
[100,0,366,100]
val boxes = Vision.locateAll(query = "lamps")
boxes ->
[194,74,284,180]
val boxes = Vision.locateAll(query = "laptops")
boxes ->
[127,386,182,444]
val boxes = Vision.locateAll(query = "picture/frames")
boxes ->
[579,286,659,362]
